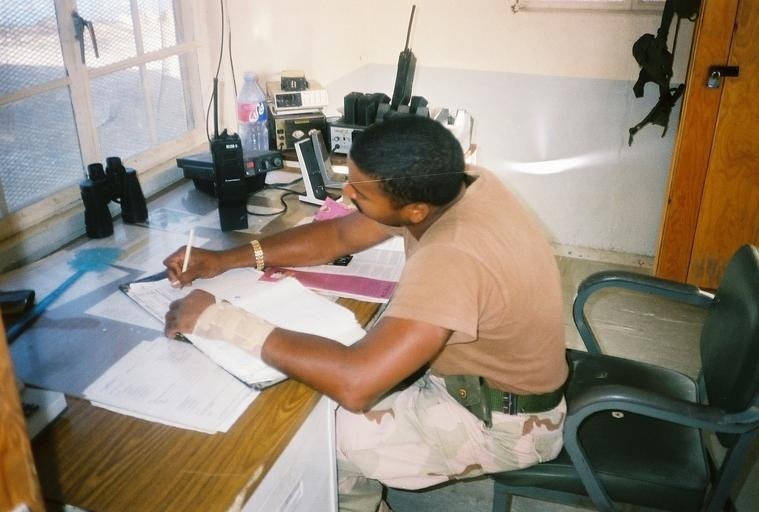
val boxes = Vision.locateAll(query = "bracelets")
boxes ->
[249,239,265,272]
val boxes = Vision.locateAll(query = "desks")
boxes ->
[9,142,477,512]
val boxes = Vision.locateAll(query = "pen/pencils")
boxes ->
[182,230,194,273]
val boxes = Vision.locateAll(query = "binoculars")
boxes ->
[79,156,148,238]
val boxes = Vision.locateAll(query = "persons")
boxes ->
[161,111,573,511]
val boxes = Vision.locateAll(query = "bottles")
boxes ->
[235,75,270,153]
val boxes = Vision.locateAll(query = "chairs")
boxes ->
[485,244,759,512]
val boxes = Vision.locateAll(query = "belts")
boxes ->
[491,389,564,413]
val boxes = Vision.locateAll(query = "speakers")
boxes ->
[311,130,349,189]
[294,138,344,206]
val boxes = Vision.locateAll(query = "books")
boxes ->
[257,192,417,309]
